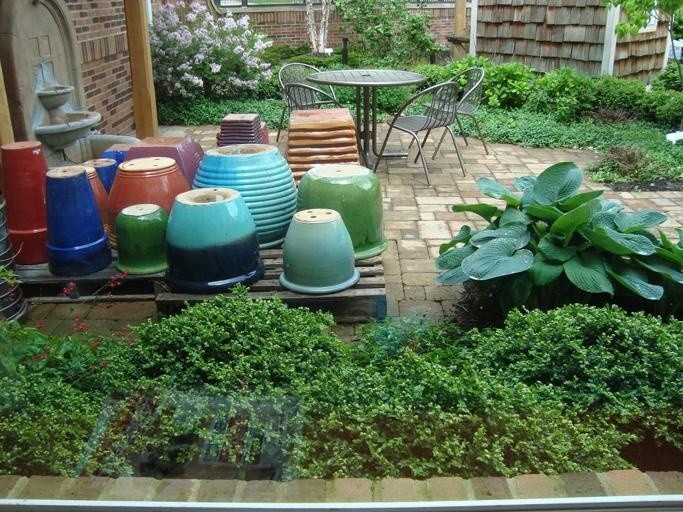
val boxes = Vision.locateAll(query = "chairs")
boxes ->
[373,80,468,188]
[408,66,489,160]
[276,62,369,170]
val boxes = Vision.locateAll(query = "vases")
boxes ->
[43,166,112,278]
[108,156,193,252]
[278,210,358,294]
[125,136,201,184]
[114,203,172,275]
[103,140,129,165]
[192,143,298,249]
[1,141,48,266]
[288,107,362,185]
[82,167,111,230]
[296,163,389,259]
[84,156,116,189]
[215,111,269,145]
[163,189,265,292]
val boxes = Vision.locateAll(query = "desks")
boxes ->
[305,68,428,171]
